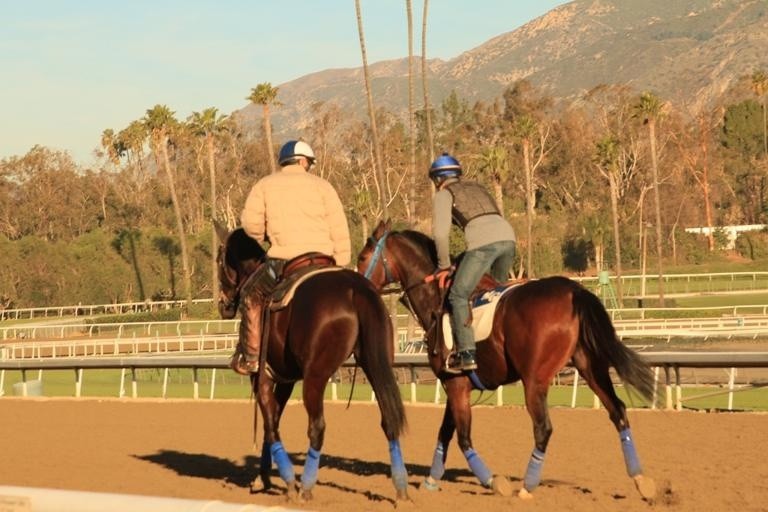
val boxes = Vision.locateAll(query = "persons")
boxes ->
[232,137,354,373]
[425,148,517,373]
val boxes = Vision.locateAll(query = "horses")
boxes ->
[357,216,666,500]
[211,217,416,509]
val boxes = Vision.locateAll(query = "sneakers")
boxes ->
[233,360,258,372]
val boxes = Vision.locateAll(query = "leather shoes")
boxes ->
[442,350,479,373]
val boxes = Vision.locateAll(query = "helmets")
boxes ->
[428,152,462,178]
[279,141,316,169]
